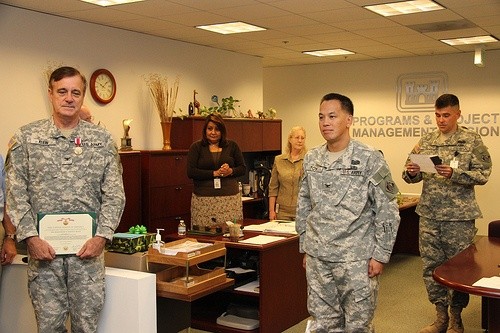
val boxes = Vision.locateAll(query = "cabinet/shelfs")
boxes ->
[166,219,311,333]
[113,151,141,234]
[140,149,195,243]
[146,237,226,295]
[167,117,283,152]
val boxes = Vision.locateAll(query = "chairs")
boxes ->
[481,220,500,333]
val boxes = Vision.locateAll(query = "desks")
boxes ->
[432,236,500,299]
[392,195,420,255]
[156,278,235,302]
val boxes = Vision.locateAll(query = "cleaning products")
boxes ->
[155,228,165,241]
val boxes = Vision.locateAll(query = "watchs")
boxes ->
[5,234,16,240]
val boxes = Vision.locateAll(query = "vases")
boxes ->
[161,121,171,149]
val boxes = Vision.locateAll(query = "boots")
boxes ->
[446,306,463,333]
[418,306,449,333]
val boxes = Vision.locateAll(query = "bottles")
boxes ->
[178,220,186,237]
[188,101,193,115]
[239,182,242,197]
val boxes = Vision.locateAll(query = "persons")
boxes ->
[187,113,245,229]
[295,92,401,333]
[0,154,17,277]
[4,67,126,333]
[268,127,308,221]
[402,93,493,333]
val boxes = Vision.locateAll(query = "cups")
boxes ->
[243,184,250,196]
[229,223,241,243]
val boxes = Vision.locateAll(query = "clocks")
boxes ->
[89,68,116,104]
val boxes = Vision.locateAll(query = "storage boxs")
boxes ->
[105,232,157,255]
[103,250,175,274]
